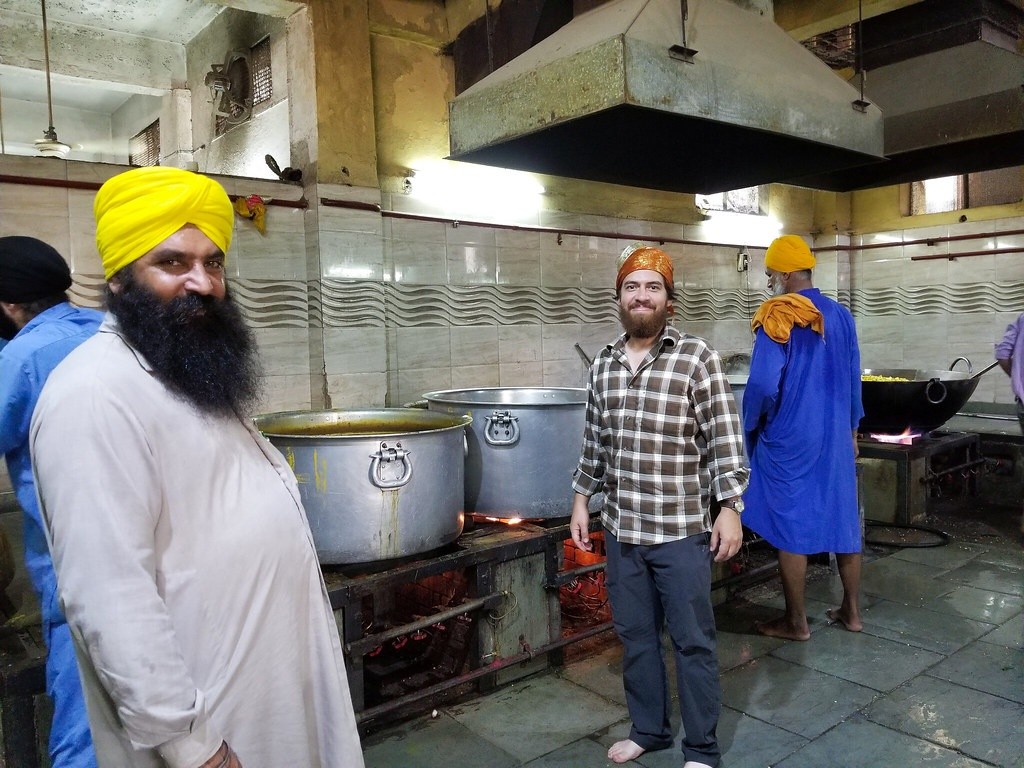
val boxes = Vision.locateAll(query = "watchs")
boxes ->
[720,497,745,514]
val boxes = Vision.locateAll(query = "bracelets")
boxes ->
[215,739,230,768]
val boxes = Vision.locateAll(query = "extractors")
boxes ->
[448,0,885,194]
[779,0,1024,193]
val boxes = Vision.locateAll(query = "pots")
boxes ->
[725,374,750,466]
[423,386,605,518]
[858,357,980,436]
[253,409,474,563]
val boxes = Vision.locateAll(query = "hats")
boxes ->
[0,236,73,303]
[764,235,816,272]
[615,248,676,288]
[92,166,235,277]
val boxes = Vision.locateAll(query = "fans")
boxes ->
[0,0,146,158]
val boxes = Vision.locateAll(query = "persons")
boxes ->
[0,235,107,768]
[22,164,367,768]
[568,245,751,768]
[740,233,867,640]
[993,311,1024,434]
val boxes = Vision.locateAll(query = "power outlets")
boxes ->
[737,254,749,272]
